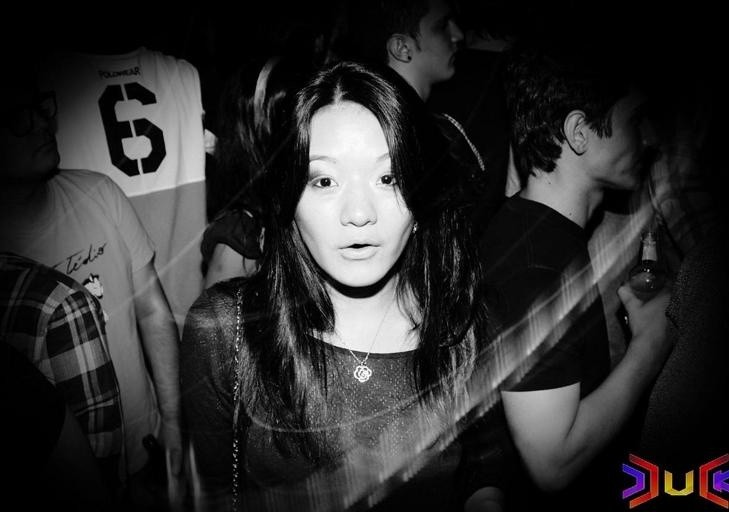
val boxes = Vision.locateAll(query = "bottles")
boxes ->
[141,433,167,483]
[628,229,666,304]
